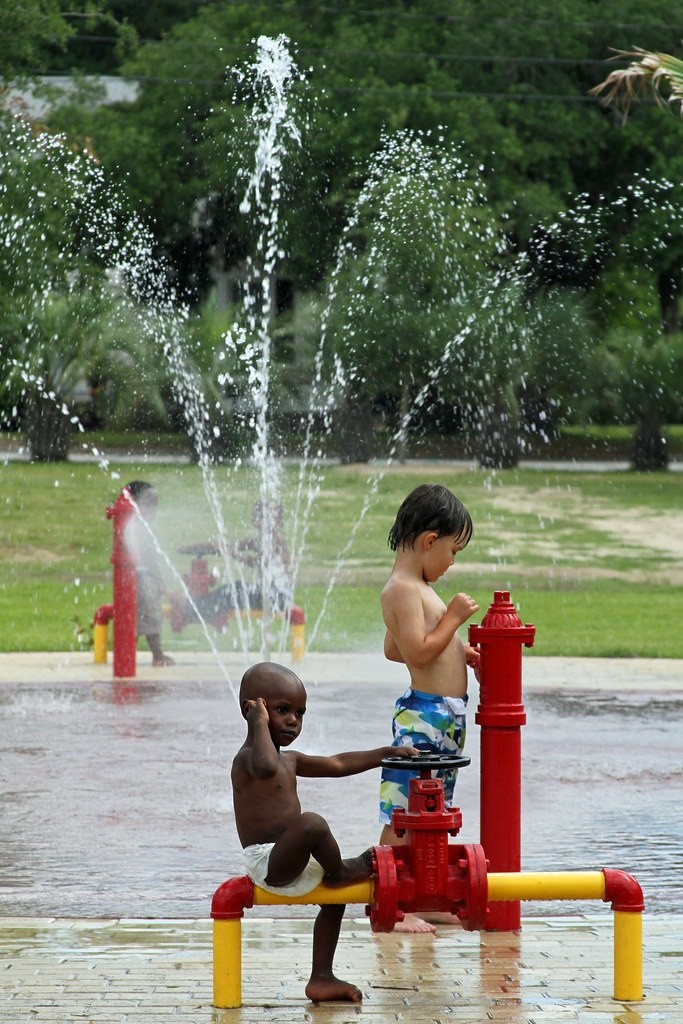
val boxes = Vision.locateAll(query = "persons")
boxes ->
[231,661,419,1006]
[122,480,291,665]
[378,483,480,935]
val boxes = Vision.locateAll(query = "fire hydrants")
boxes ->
[106,483,145,678]
[469,591,537,932]
